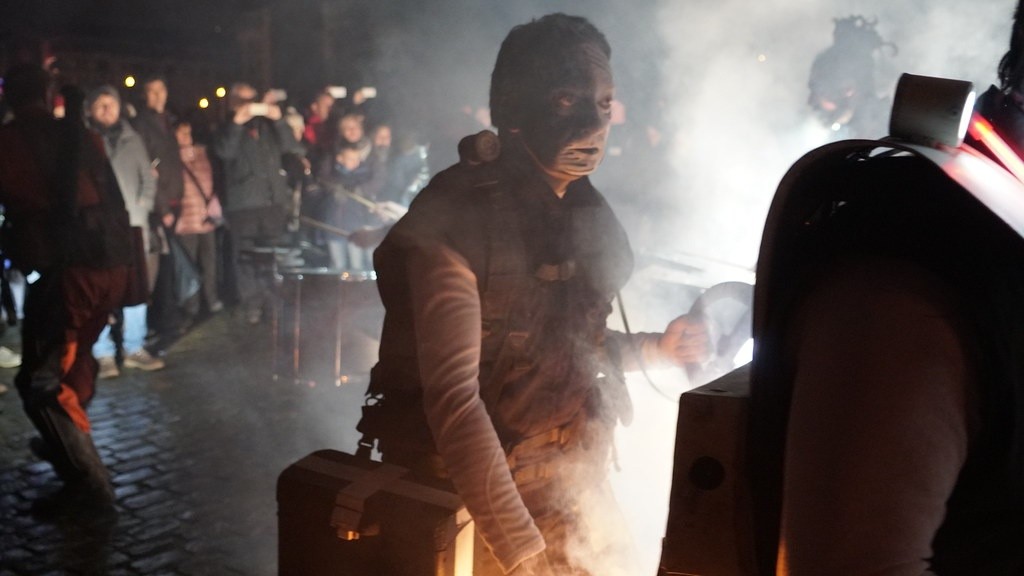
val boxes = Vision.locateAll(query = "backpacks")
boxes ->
[149,213,200,336]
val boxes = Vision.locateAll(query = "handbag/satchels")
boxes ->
[277,450,474,576]
[206,197,226,228]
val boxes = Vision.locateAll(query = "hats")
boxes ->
[89,84,122,111]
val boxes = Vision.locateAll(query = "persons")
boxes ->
[1,70,427,376]
[743,0,1024,576]
[0,64,131,526]
[804,18,895,147]
[380,14,714,576]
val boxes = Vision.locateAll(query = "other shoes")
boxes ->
[32,489,95,514]
[29,435,59,468]
[0,346,23,368]
[97,357,119,379]
[123,349,164,371]
